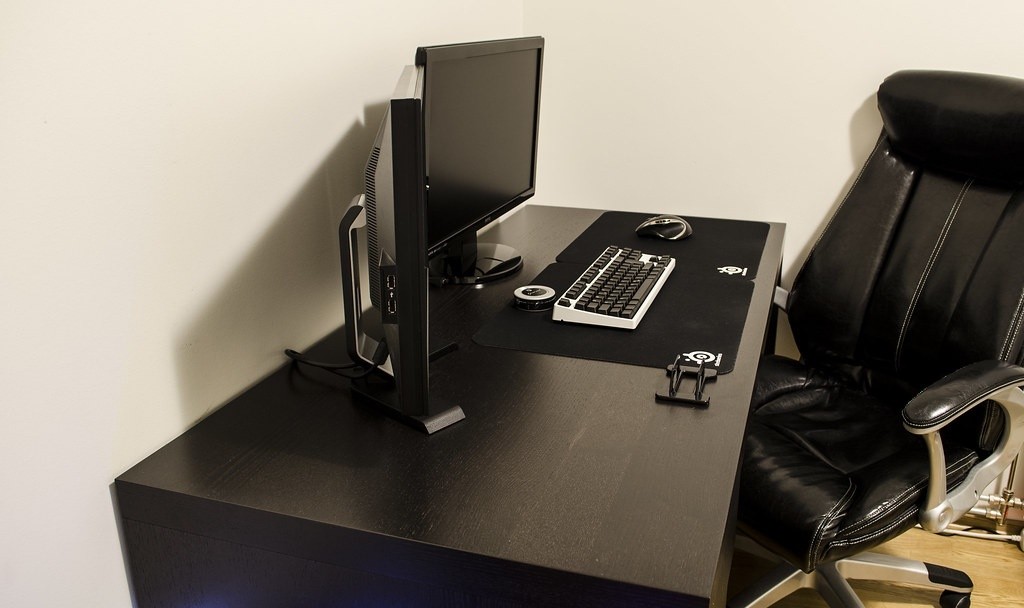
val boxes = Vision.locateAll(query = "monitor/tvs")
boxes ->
[416,36,544,284]
[338,99,466,435]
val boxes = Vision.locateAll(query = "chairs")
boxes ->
[728,69,1024,608]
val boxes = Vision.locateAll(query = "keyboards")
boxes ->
[552,244,677,329]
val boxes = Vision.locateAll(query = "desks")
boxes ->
[112,205,787,608]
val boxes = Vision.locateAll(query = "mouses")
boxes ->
[635,215,692,240]
